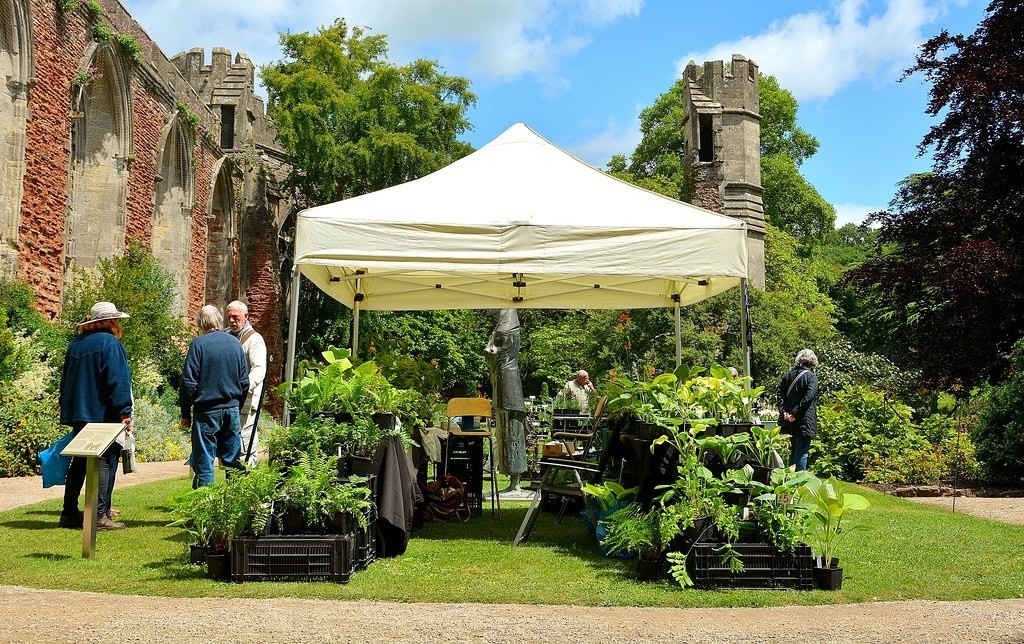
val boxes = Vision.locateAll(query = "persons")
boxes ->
[727,367,739,381]
[560,369,597,416]
[217,299,267,469]
[59,300,136,531]
[180,305,250,491]
[777,348,820,472]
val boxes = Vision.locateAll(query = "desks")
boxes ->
[540,449,602,517]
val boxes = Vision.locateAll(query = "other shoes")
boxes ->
[94,514,126,531]
[109,507,121,515]
[61,511,84,528]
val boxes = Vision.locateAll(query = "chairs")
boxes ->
[513,409,628,546]
[444,397,502,521]
[550,393,609,528]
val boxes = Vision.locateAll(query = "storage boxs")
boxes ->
[543,442,575,456]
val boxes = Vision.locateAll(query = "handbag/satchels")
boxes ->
[40,429,73,487]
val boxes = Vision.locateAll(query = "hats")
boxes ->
[79,301,130,324]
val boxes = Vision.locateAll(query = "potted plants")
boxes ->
[162,344,873,591]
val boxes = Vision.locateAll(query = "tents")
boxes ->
[281,122,751,431]
